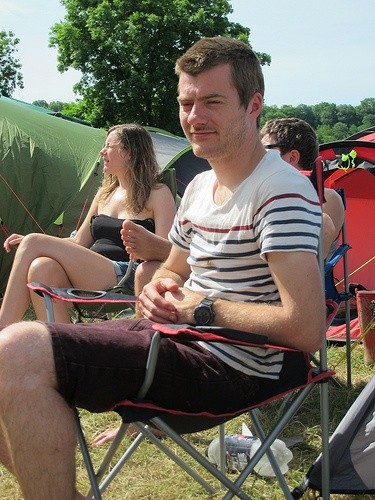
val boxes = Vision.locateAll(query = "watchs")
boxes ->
[193,296,219,325]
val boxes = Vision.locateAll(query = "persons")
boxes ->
[0,35,326,500]
[0,123,177,332]
[91,118,346,448]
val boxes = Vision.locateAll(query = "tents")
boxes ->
[1,95,375,345]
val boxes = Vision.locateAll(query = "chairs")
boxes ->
[25,155,331,500]
[321,186,352,392]
[69,166,183,313]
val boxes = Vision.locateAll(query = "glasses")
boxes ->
[264,143,285,150]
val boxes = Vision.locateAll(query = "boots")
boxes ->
[355,288,375,365]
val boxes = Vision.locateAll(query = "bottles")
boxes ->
[205,435,293,476]
